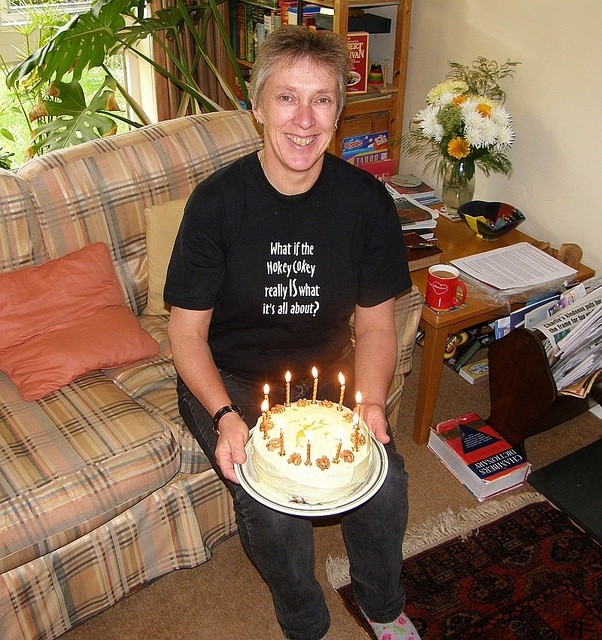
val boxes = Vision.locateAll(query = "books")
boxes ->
[412,322,491,388]
[342,130,388,166]
[229,0,333,63]
[354,161,444,272]
[347,31,369,94]
[490,277,602,410]
[449,241,578,298]
[426,412,532,503]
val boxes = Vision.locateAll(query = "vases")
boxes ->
[442,159,477,214]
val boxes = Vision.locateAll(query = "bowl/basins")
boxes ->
[458,200,526,240]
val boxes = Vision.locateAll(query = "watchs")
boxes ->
[212,403,244,436]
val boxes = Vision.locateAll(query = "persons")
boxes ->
[163,23,416,489]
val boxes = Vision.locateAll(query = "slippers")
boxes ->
[359,606,421,640]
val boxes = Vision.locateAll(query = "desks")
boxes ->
[407,200,598,445]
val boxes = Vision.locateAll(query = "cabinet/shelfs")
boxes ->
[226,0,411,185]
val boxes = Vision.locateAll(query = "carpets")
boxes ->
[326,489,600,637]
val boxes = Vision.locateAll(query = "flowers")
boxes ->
[390,55,521,193]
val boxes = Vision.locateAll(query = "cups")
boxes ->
[426,264,467,311]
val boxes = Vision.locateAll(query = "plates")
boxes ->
[234,426,389,517]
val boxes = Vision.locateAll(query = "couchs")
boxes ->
[0,108,425,640]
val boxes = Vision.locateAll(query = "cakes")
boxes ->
[249,399,375,507]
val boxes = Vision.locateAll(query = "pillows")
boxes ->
[143,196,190,315]
[0,240,161,404]
